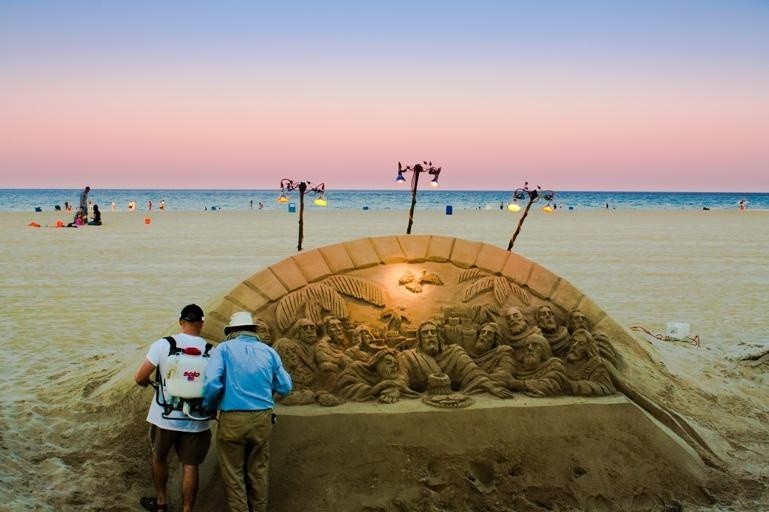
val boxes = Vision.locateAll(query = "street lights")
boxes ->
[506,183,554,253]
[396,161,442,234]
[279,178,329,251]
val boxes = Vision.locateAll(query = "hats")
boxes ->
[181,304,204,322]
[224,312,260,336]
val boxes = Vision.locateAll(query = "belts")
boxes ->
[220,408,272,413]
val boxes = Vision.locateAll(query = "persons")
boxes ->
[73,206,87,224]
[86,203,103,226]
[249,303,621,406]
[79,185,90,215]
[133,301,217,512]
[201,310,292,512]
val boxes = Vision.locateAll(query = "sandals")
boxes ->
[140,496,168,512]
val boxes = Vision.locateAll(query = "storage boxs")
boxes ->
[666,321,690,339]
